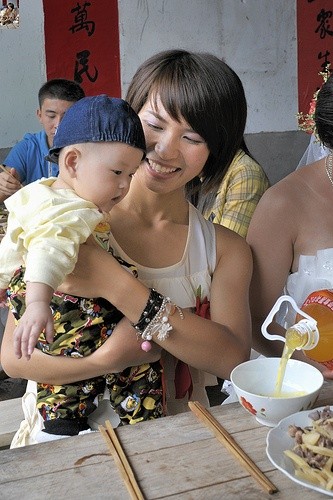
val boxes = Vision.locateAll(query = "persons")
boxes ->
[0,79,85,205]
[246,60,333,379]
[0,93,165,446]
[185,136,271,240]
[10,48,254,449]
[0,0,19,29]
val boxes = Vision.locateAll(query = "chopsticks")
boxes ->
[0,162,24,187]
[98,419,145,500]
[187,400,279,494]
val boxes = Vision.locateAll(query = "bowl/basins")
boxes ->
[231,357,324,428]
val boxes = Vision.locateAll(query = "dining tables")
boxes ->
[0,381,333,500]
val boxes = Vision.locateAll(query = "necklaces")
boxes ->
[325,153,333,185]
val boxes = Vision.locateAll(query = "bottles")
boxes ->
[286,288,333,372]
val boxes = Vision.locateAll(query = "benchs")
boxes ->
[0,397,23,449]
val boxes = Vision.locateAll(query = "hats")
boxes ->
[44,94,148,163]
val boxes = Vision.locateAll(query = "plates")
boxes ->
[265,404,333,497]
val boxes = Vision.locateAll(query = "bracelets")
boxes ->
[128,285,184,351]
